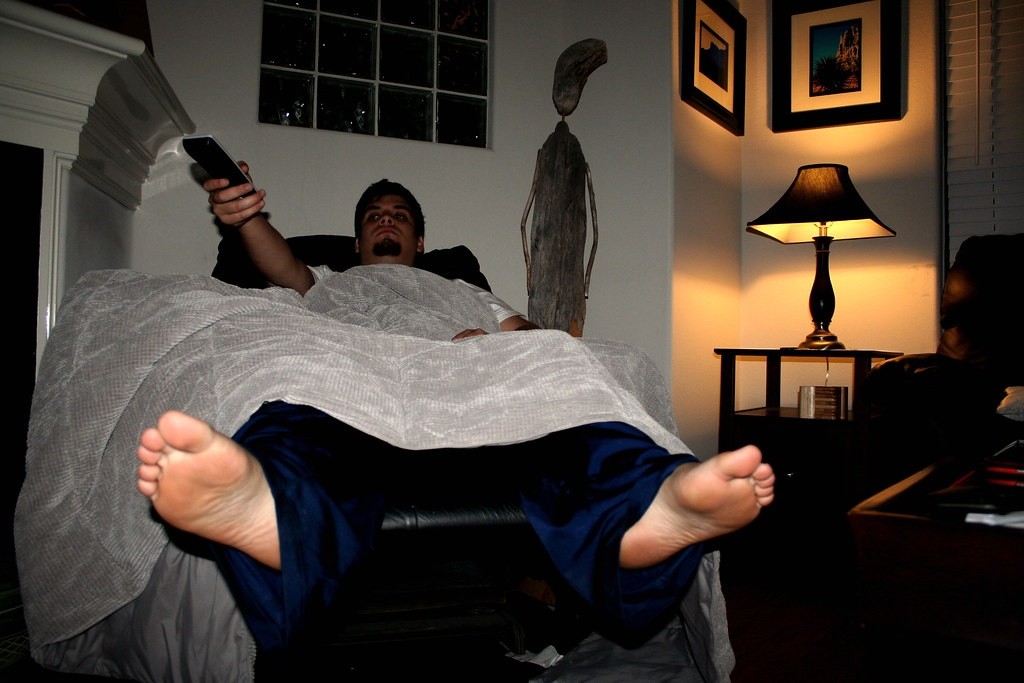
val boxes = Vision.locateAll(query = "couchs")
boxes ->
[815,231,1024,633]
[196,235,552,654]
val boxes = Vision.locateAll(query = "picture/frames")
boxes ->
[680,0,747,137]
[771,0,902,134]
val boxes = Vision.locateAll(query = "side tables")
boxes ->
[706,345,906,612]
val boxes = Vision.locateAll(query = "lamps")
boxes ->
[745,162,898,351]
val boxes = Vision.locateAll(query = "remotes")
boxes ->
[182,133,264,201]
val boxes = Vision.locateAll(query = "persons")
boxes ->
[136,159,778,654]
